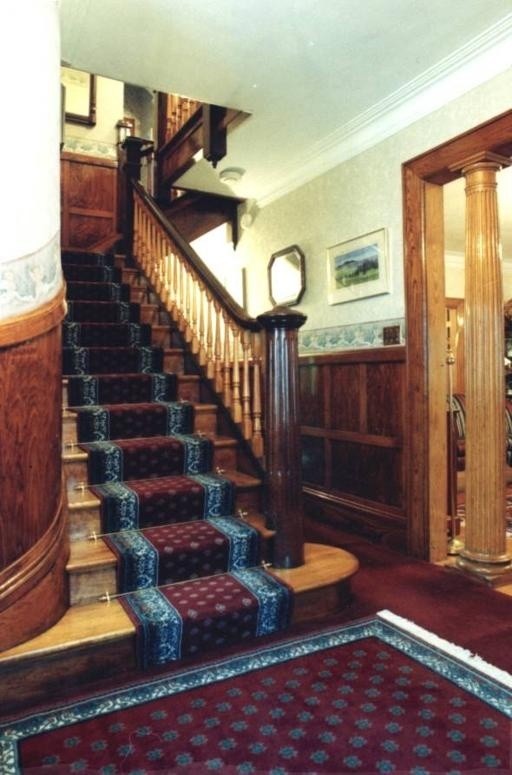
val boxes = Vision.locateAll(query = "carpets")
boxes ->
[1,610,512,775]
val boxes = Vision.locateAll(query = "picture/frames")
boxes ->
[325,227,391,307]
[60,66,98,128]
[220,266,248,313]
[266,243,306,309]
